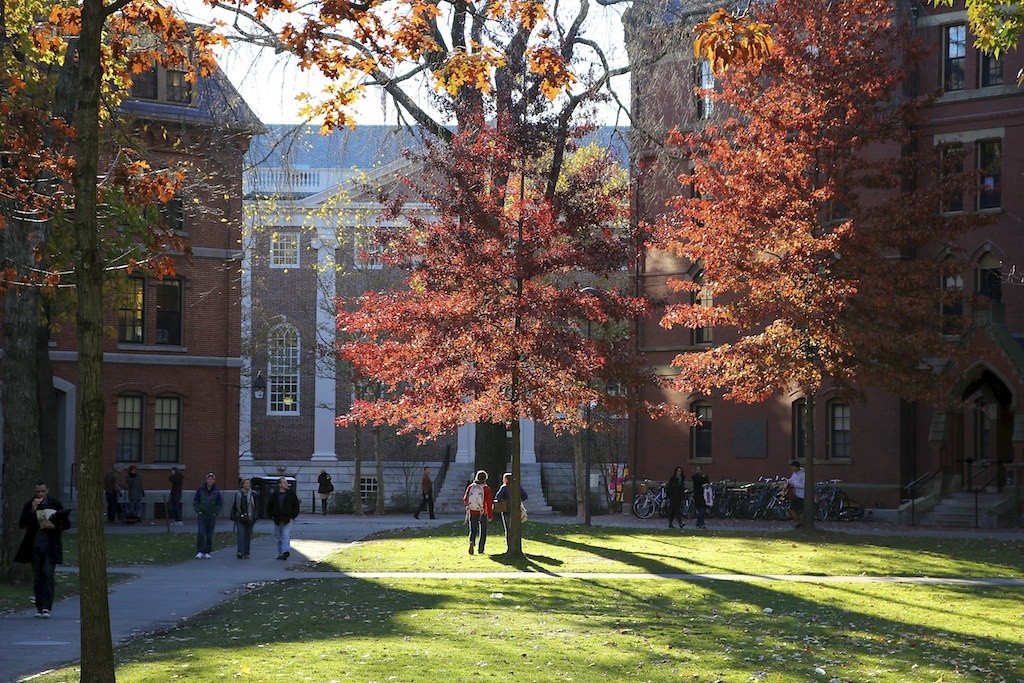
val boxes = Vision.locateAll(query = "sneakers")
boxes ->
[196,552,203,558]
[206,553,210,559]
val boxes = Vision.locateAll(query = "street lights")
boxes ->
[580,285,598,524]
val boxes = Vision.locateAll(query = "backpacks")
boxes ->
[470,482,485,512]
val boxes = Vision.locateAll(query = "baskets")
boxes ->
[638,484,647,494]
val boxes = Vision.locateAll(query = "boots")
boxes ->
[322,499,326,516]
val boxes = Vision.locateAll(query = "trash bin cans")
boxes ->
[250,476,296,521]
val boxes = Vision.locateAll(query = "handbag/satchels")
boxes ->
[238,512,250,523]
[493,499,510,513]
[704,485,714,506]
[787,484,797,501]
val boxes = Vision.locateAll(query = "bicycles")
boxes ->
[632,474,867,522]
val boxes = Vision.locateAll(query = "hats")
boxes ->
[790,461,800,467]
[129,464,136,470]
[206,472,216,479]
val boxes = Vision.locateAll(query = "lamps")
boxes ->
[252,375,267,400]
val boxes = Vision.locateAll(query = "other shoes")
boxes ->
[237,552,243,559]
[171,521,183,526]
[277,556,283,559]
[245,554,249,559]
[283,552,289,560]
[35,611,42,617]
[42,609,49,617]
[469,541,474,555]
[669,522,706,529]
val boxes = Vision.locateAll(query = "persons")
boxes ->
[495,471,528,548]
[230,477,258,558]
[413,467,435,520]
[665,465,686,529]
[691,465,712,528]
[785,460,806,529]
[267,475,300,560]
[193,471,222,559]
[14,481,73,619]
[103,463,125,524]
[317,468,334,517]
[166,466,185,526]
[463,470,494,554]
[124,464,145,520]
